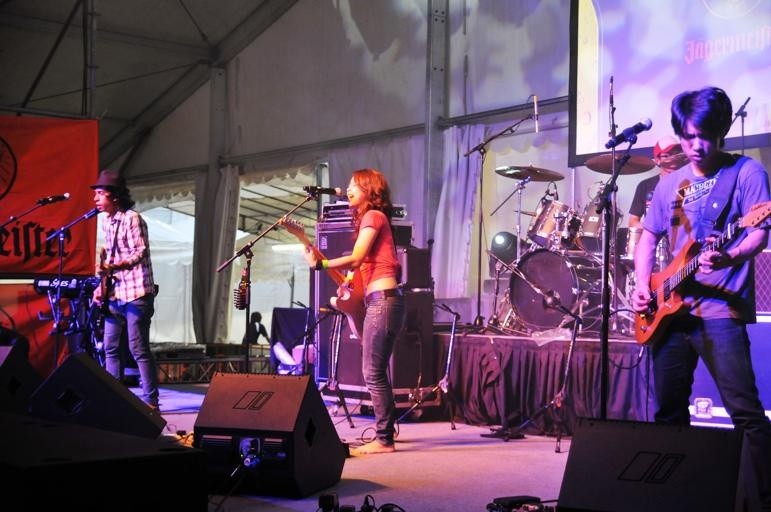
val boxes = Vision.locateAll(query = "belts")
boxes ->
[366,288,403,304]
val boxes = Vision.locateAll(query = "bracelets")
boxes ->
[315,259,322,269]
[321,259,330,269]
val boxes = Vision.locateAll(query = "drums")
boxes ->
[525,199,572,247]
[573,200,624,257]
[509,249,606,332]
[615,227,643,264]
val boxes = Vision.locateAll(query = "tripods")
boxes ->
[292,301,355,427]
[397,303,460,429]
[453,113,634,337]
[485,247,583,452]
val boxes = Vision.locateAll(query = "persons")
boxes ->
[90,170,162,419]
[631,85,771,430]
[302,168,406,454]
[243,311,273,344]
[627,135,682,227]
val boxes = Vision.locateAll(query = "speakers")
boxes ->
[194,371,345,501]
[555,417,771,512]
[0,345,208,512]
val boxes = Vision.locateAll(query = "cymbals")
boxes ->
[586,153,656,175]
[494,165,564,181]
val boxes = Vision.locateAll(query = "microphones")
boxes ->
[605,118,652,149]
[303,186,342,197]
[35,193,70,205]
[85,207,101,219]
[534,95,539,133]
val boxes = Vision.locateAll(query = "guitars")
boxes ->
[635,201,771,344]
[279,218,367,341]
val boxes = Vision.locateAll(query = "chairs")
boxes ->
[145,340,272,388]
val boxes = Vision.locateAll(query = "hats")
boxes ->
[653,134,682,160]
[89,169,129,189]
